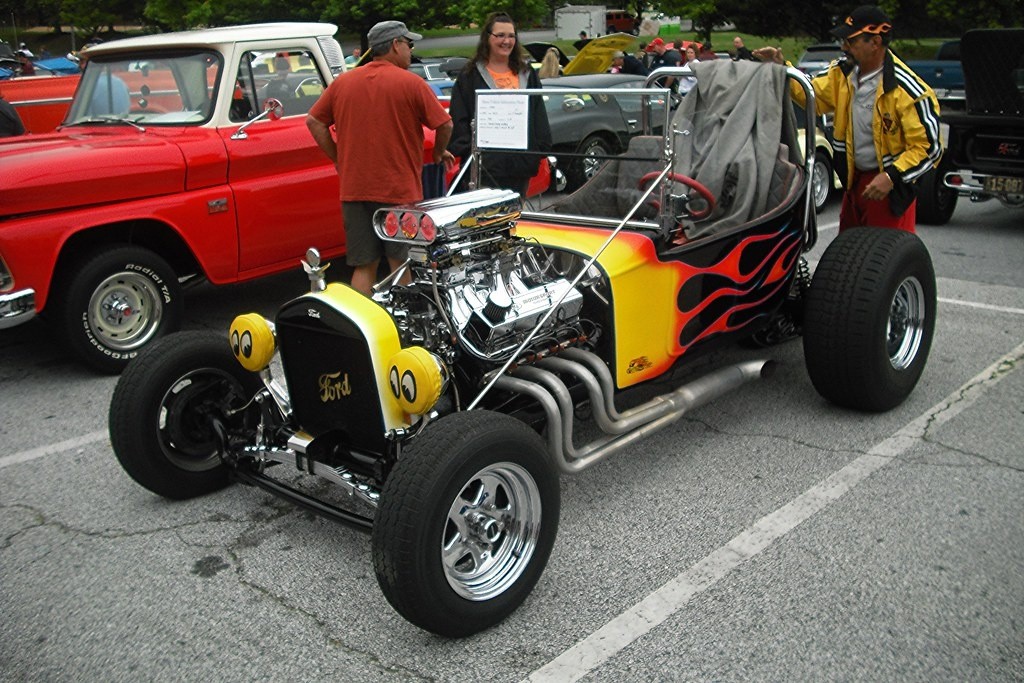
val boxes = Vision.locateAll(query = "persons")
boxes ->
[752,5,944,239]
[306,21,454,302]
[446,13,554,210]
[539,31,758,100]
[0,42,422,139]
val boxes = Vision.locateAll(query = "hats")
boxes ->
[72,44,99,62]
[612,51,625,61]
[649,38,665,48]
[20,43,25,48]
[834,5,893,40]
[368,20,423,48]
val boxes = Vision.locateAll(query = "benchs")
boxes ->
[551,133,797,236]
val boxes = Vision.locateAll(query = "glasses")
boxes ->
[837,35,877,48]
[391,37,415,48]
[488,30,519,40]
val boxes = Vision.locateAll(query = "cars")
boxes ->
[0,26,1024,227]
[109,66,938,643]
[1,20,550,375]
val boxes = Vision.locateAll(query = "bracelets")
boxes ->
[885,172,894,187]
